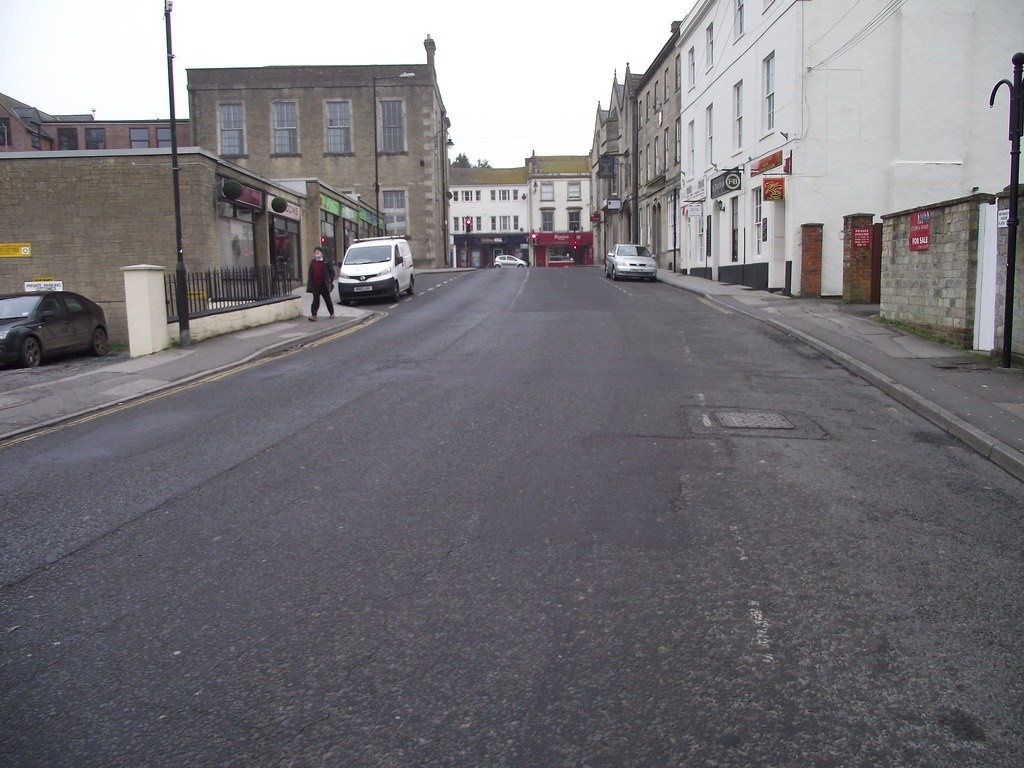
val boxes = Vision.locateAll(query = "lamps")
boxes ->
[718,199,725,211]
[656,202,661,211]
[435,130,454,149]
[533,180,539,194]
[652,204,656,212]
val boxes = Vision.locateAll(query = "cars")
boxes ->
[605,243,657,281]
[493,254,529,269]
[0,291,111,369]
[547,256,575,265]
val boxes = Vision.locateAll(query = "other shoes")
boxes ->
[330,313,335,318]
[309,315,317,322]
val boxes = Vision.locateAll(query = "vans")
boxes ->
[336,235,416,306]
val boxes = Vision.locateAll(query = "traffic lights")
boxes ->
[465,219,471,233]
[531,234,537,245]
[572,239,578,251]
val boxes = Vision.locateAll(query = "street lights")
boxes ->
[571,222,579,265]
[527,181,539,267]
[373,71,415,237]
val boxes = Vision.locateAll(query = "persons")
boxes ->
[306,246,336,322]
